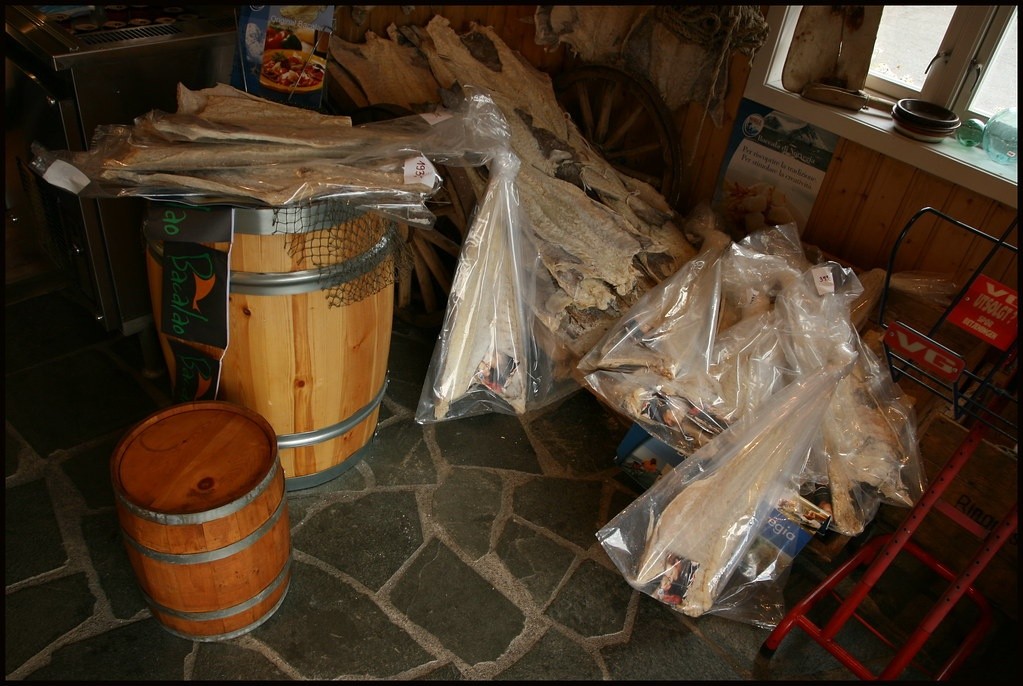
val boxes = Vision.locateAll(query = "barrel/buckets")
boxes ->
[144,199,393,494]
[111,402,289,641]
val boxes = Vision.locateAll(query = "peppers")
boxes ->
[265,27,303,52]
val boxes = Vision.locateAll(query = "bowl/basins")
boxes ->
[897,99,959,126]
[891,113,956,142]
[892,105,961,132]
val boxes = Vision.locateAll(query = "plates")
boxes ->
[258,49,327,92]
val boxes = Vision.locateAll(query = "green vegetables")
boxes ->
[271,50,289,62]
